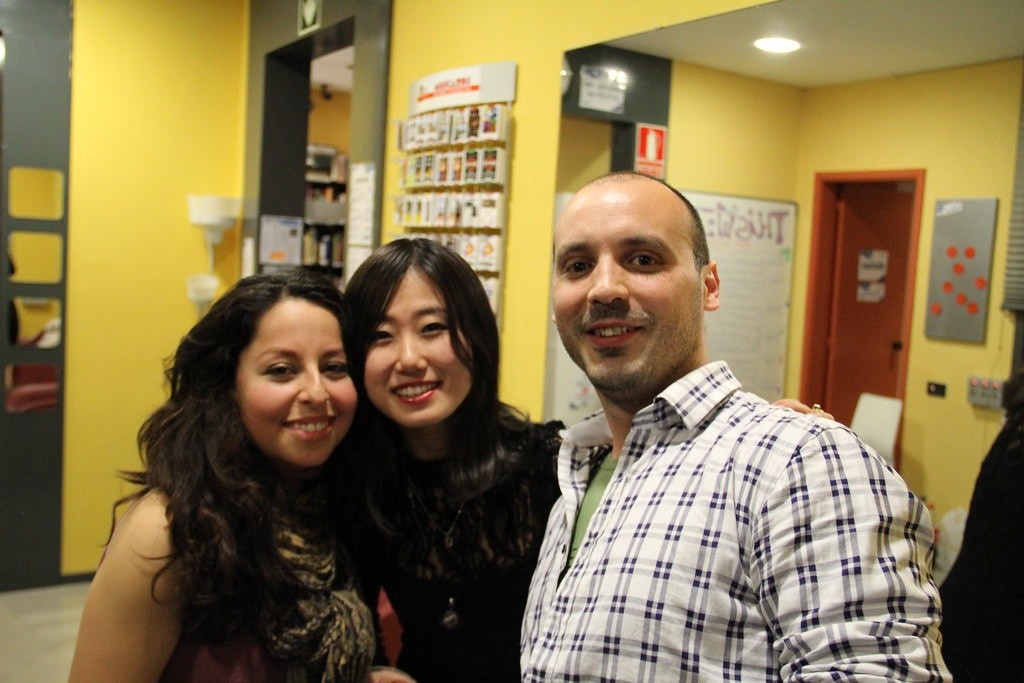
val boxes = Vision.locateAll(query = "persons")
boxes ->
[67,276,402,678]
[512,170,955,683]
[938,314,1024,683]
[289,237,562,682]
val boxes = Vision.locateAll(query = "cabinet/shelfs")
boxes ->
[303,175,345,290]
